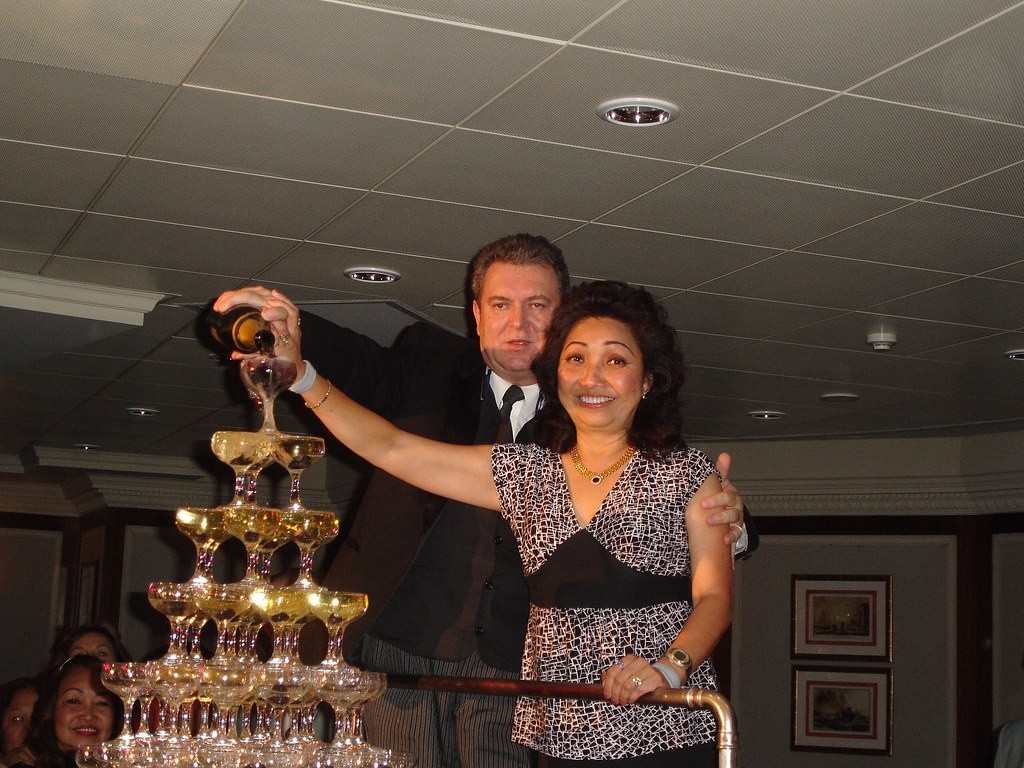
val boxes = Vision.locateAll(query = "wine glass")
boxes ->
[76,357,416,768]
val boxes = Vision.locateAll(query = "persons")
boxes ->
[213,232,760,768]
[0,568,360,768]
[259,289,735,768]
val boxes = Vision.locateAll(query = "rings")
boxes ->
[297,317,301,326]
[730,523,743,534]
[277,333,291,347]
[613,658,624,671]
[628,673,642,686]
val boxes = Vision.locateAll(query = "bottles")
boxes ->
[208,297,275,352]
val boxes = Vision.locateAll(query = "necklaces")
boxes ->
[569,441,636,485]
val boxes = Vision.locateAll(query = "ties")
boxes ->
[495,385,526,445]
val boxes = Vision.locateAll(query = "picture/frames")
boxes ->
[789,665,895,756]
[77,560,99,628]
[788,574,895,663]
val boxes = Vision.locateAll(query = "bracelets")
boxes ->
[287,359,317,394]
[652,661,681,689]
[304,378,331,409]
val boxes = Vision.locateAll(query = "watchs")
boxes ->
[664,648,694,685]
